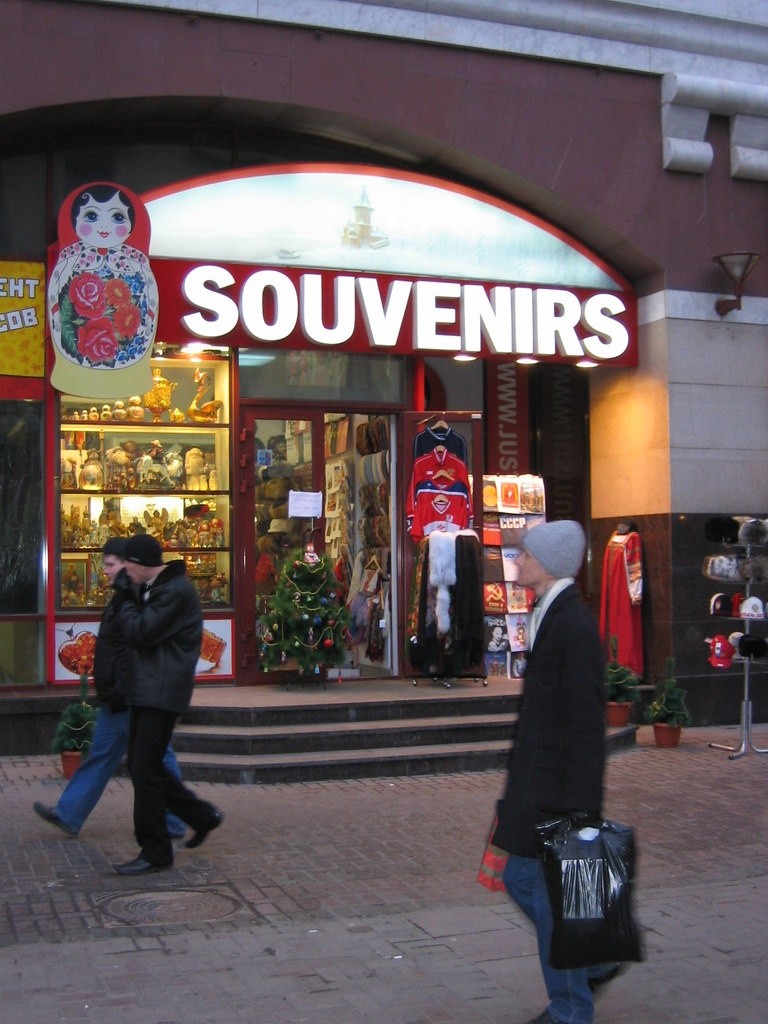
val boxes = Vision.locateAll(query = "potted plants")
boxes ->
[604,636,642,727]
[50,671,101,780]
[642,656,694,748]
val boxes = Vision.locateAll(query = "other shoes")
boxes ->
[588,962,620,992]
[524,1011,567,1024]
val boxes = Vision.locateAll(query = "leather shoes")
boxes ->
[185,810,222,848]
[111,853,174,875]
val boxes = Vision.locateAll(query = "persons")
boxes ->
[489,520,625,1024]
[32,537,187,840]
[112,534,224,875]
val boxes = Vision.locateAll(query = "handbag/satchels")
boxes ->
[533,814,645,968]
[476,799,510,892]
[51,673,102,761]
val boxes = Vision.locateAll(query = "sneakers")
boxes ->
[33,801,79,837]
[171,837,184,848]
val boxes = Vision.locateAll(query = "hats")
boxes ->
[124,534,163,566]
[103,537,129,555]
[254,460,304,584]
[698,514,768,671]
[523,519,585,579]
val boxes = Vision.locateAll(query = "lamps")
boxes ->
[712,248,762,316]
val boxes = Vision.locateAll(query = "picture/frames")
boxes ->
[61,559,91,597]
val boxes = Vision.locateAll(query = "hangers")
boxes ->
[434,491,448,504]
[431,413,448,430]
[436,438,445,452]
[366,587,385,610]
[363,555,383,574]
[432,461,454,481]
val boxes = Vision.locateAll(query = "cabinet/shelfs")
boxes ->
[55,351,233,612]
